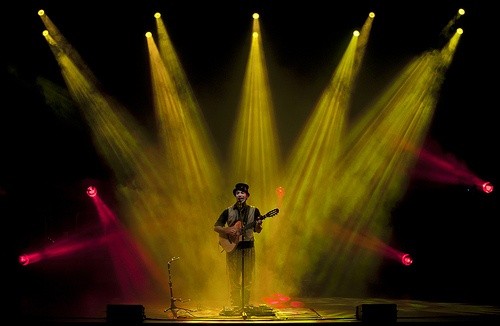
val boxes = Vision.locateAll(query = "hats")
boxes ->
[232,183,250,197]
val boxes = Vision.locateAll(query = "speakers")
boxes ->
[355,303,399,323]
[104,303,147,324]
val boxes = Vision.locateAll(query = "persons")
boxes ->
[214,184,263,307]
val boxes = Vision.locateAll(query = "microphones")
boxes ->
[237,198,242,208]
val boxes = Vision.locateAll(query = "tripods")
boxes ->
[218,208,276,319]
[162,257,197,313]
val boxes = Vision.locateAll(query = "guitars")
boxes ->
[218,208,281,253]
